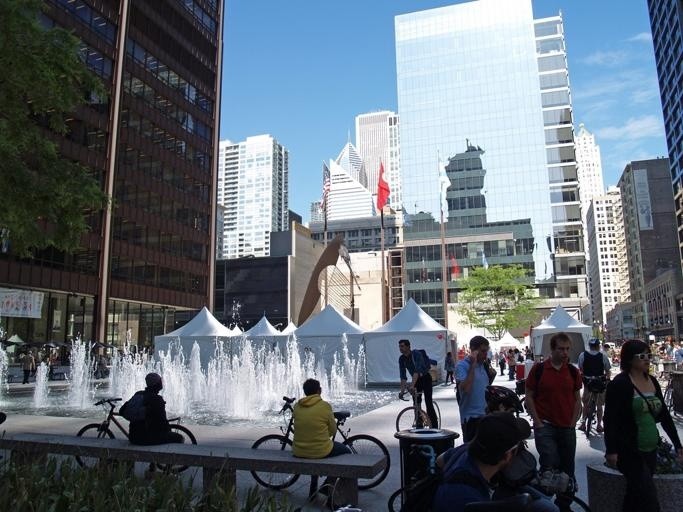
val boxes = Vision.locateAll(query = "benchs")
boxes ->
[49,372,66,381]
[0,374,14,382]
[0,432,387,512]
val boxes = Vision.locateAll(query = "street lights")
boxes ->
[593,320,600,339]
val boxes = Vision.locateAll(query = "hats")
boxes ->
[146,373,161,387]
[475,412,531,453]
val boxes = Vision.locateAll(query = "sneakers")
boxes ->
[412,416,425,427]
[319,482,333,496]
[579,425,586,430]
[145,469,180,480]
[597,427,604,432]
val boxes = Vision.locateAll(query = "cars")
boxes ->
[604,340,622,367]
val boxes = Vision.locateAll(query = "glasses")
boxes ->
[634,353,653,361]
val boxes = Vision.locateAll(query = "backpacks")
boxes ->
[124,394,146,421]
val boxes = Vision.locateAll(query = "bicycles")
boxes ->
[659,370,675,419]
[248,396,390,491]
[395,386,441,432]
[294,478,363,512]
[386,476,591,512]
[516,374,610,435]
[73,397,196,474]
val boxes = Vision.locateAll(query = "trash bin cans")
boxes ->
[394,429,460,512]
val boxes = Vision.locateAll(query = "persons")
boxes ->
[122,373,184,479]
[305,347,315,368]
[293,378,352,507]
[19,349,55,385]
[399,332,683,511]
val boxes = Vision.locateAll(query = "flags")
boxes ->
[378,159,390,211]
[451,254,459,278]
[481,247,488,270]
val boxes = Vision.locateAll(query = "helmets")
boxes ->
[484,385,524,413]
[589,337,600,345]
